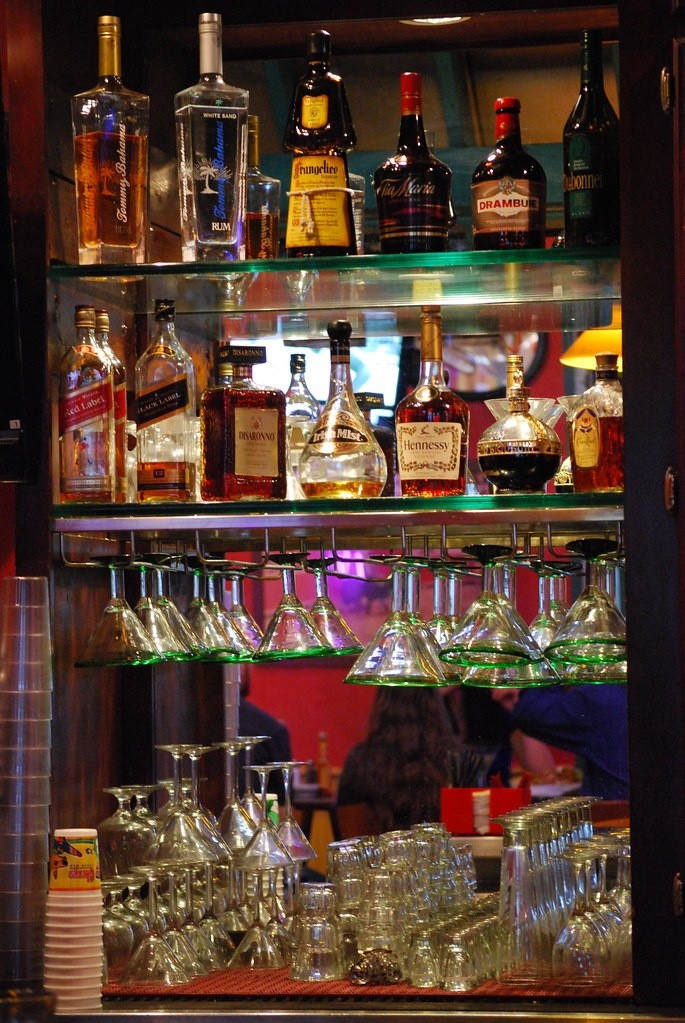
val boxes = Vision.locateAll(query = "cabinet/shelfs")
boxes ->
[46,241,626,553]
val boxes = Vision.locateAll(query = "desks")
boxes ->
[291,795,342,879]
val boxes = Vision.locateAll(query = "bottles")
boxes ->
[282,30,357,257]
[72,14,151,283]
[470,98,546,253]
[349,174,365,256]
[373,69,453,253]
[247,114,282,262]
[316,732,332,798]
[175,13,247,282]
[562,28,622,248]
[58,301,628,496]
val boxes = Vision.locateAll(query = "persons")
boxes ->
[336,676,627,892]
[239,662,331,890]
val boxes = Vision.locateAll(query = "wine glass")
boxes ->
[74,536,627,687]
[97,734,315,986]
[490,793,631,986]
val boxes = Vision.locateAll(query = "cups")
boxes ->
[45,828,105,1011]
[290,823,497,995]
[0,576,54,1003]
[485,398,556,427]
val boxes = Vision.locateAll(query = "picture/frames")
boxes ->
[404,329,547,402]
